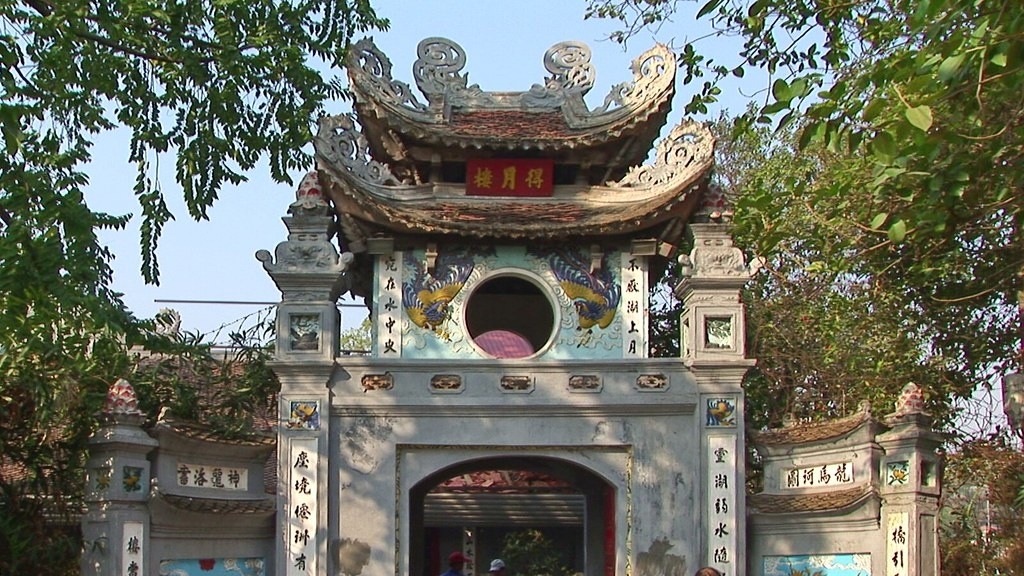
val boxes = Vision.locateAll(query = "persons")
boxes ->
[695,567,721,576]
[440,551,473,576]
[489,558,507,576]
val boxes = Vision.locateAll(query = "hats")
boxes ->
[489,559,506,571]
[448,551,473,563]
